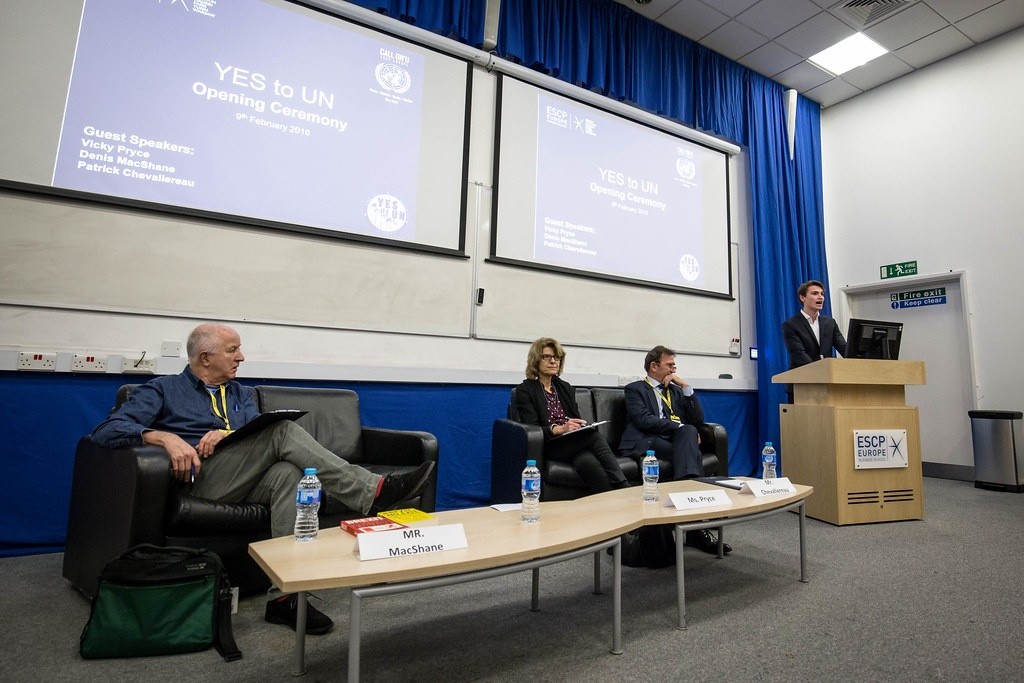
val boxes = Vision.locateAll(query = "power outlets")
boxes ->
[18,352,58,371]
[72,353,109,373]
[121,357,159,374]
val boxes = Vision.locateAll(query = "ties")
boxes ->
[657,383,671,421]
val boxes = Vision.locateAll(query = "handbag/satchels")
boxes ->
[77,546,244,662]
[620,527,677,570]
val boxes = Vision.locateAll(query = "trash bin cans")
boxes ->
[968,409,1024,493]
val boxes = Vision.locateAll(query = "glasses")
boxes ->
[542,353,564,361]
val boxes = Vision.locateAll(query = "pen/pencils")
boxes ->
[191,463,194,483]
[565,416,586,427]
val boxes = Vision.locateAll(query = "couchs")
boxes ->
[62,384,440,600]
[489,387,729,504]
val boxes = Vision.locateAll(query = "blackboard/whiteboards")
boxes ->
[472,183,742,358]
[1,179,475,340]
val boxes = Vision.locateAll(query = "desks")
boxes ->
[248,476,815,683]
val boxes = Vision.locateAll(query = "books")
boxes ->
[378,508,438,528]
[339,515,409,537]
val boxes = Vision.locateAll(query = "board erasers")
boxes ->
[477,288,485,305]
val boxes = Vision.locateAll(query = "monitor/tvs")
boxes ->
[845,318,903,360]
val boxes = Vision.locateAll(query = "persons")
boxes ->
[617,345,733,555]
[782,279,847,406]
[88,323,437,636]
[514,333,645,565]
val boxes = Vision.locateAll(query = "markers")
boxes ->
[732,338,740,348]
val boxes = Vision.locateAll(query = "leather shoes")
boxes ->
[686,527,732,554]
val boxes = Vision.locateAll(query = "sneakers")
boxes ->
[368,460,436,516]
[265,589,335,635]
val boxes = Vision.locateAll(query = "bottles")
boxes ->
[521,460,541,524]
[642,450,659,503]
[762,442,776,480]
[294,468,322,542]
